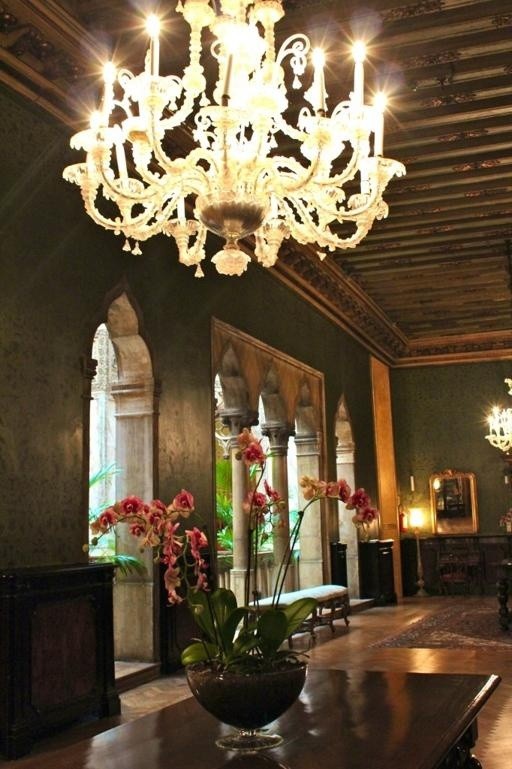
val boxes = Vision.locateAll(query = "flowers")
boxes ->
[74,427,380,670]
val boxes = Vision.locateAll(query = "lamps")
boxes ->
[408,508,429,598]
[484,376,512,452]
[56,1,408,279]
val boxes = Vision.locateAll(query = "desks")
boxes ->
[2,666,500,769]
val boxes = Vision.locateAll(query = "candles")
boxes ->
[504,474,508,484]
[409,475,416,491]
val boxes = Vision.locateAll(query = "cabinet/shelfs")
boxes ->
[329,539,398,606]
[401,534,512,596]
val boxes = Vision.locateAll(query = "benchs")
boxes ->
[246,586,352,650]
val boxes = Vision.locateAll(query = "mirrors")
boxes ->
[428,468,479,535]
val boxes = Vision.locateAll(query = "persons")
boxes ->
[446,482,463,511]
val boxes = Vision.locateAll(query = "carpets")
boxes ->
[369,602,511,652]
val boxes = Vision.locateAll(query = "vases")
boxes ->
[181,656,309,753]
